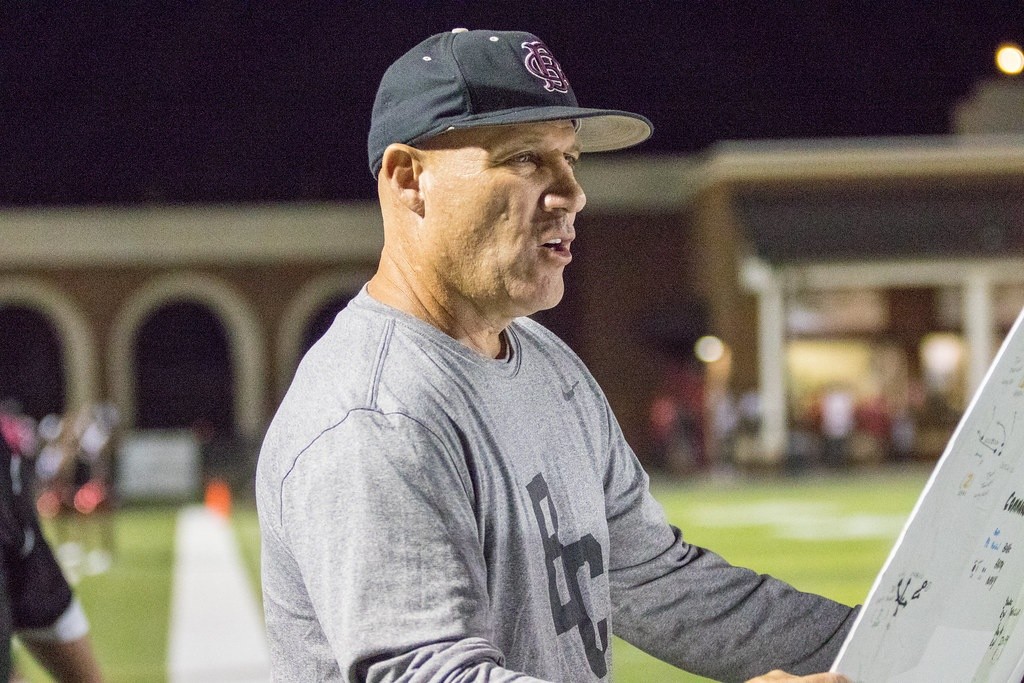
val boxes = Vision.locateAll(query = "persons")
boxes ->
[0,426,105,683]
[254,25,863,683]
[809,379,899,470]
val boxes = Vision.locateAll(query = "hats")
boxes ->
[367,28,654,181]
